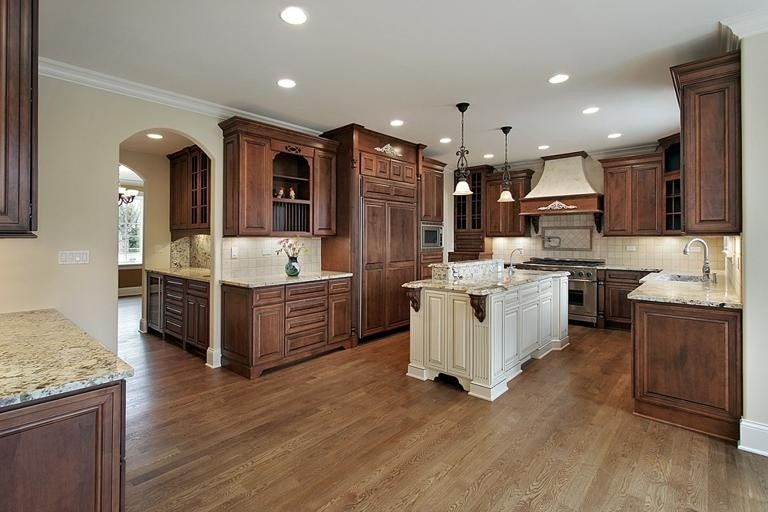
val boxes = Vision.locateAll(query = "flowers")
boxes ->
[275,239,308,262]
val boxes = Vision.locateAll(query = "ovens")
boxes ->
[568,281,597,328]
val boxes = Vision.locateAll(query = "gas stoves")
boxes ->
[516,257,605,282]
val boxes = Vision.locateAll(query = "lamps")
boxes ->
[496,125,516,204]
[452,102,474,196]
[597,153,667,239]
[118,186,139,207]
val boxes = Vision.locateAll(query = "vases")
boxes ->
[284,257,301,277]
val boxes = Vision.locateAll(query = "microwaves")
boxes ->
[421,224,443,249]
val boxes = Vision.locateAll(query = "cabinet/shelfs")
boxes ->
[422,157,447,228]
[147,271,210,362]
[321,122,424,341]
[401,267,571,402]
[484,166,535,238]
[597,271,658,329]
[217,115,340,237]
[0,379,127,512]
[454,165,495,251]
[219,276,351,379]
[166,144,211,241]
[669,47,742,236]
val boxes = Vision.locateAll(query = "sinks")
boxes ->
[503,271,546,282]
[639,274,715,282]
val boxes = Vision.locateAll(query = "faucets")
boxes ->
[505,248,522,278]
[684,237,711,281]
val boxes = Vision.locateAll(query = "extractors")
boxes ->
[519,150,603,216]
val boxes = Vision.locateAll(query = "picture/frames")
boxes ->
[0,0,38,239]
